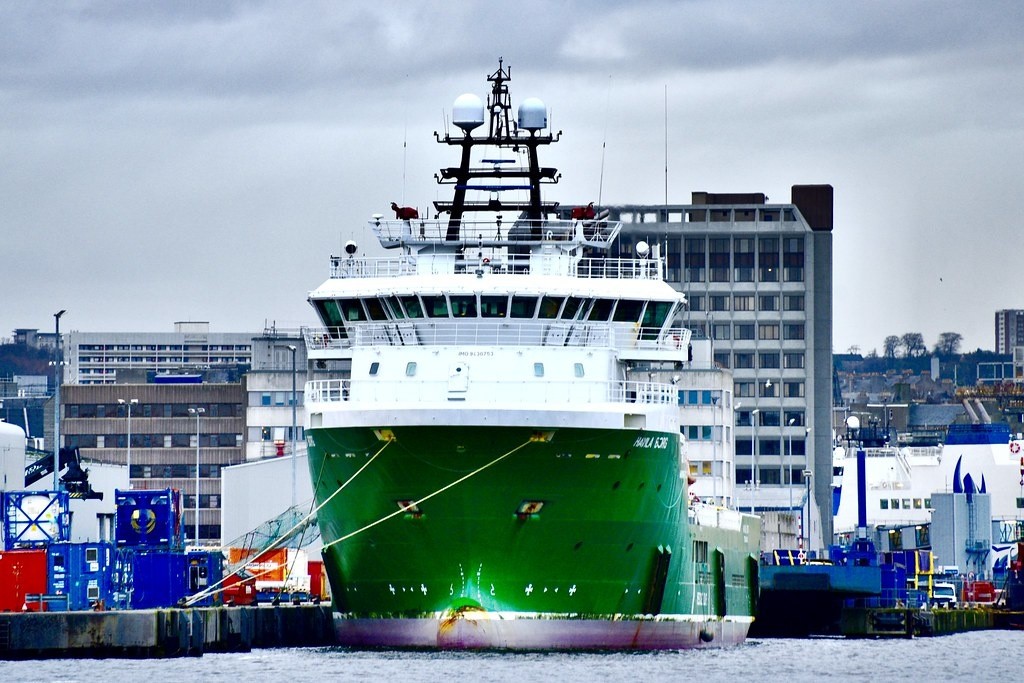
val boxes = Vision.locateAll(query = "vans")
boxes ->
[931,583,957,609]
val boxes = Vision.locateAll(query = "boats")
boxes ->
[299,58,760,651]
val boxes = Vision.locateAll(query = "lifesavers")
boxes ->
[673,336,680,348]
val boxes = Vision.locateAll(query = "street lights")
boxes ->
[54,310,67,491]
[117,398,138,489]
[802,469,812,565]
[751,409,760,516]
[789,419,795,514]
[285,345,298,549]
[188,408,205,547]
